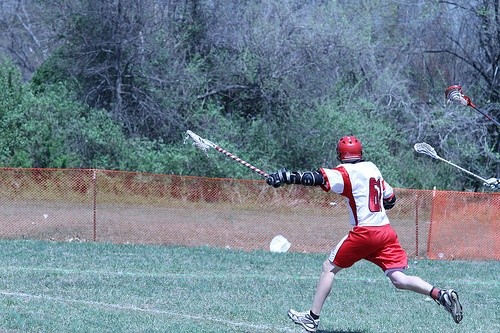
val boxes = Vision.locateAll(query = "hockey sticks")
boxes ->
[184,129,269,182]
[444,85,500,131]
[413,141,498,188]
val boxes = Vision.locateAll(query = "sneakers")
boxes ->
[437,287,463,324]
[287,308,319,332]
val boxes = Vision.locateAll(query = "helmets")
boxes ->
[337,135,362,162]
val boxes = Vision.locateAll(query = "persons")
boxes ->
[483,177,500,190]
[267,136,463,333]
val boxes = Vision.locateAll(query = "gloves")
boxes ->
[266,171,281,188]
[483,178,500,192]
